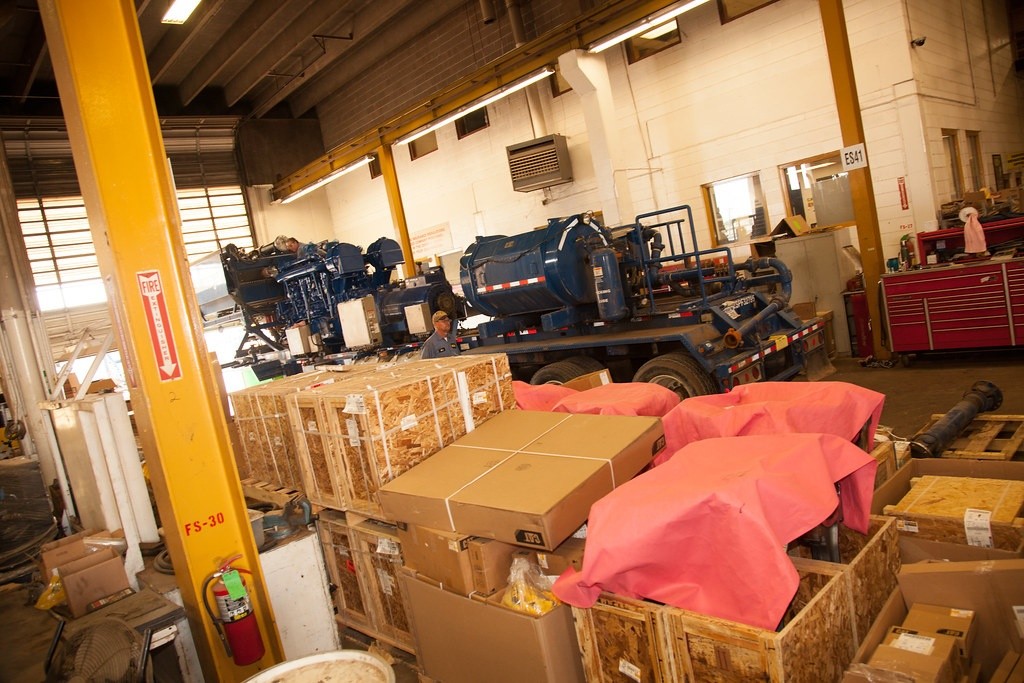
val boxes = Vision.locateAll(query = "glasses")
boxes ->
[439,319,453,323]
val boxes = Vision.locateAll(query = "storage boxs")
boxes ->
[35,353,1024,683]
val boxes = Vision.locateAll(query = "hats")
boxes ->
[432,310,448,324]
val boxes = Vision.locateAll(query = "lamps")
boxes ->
[586,0,711,55]
[280,156,375,204]
[393,66,556,145]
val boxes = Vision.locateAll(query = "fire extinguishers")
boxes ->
[897,231,917,268]
[203,554,266,667]
[851,272,863,291]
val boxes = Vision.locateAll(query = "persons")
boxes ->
[420,311,461,359]
